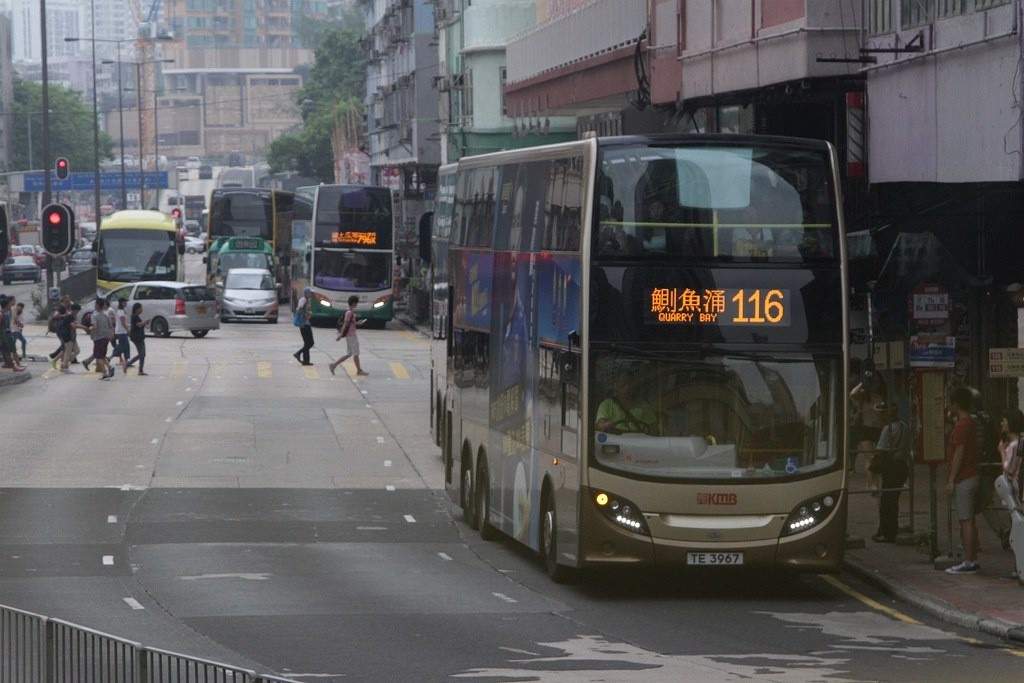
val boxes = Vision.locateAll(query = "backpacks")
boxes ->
[336,309,354,337]
[81,309,97,327]
[976,412,1003,478]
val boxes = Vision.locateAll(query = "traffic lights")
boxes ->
[41,204,71,257]
[55,157,69,180]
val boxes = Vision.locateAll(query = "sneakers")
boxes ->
[945,561,976,574]
[999,571,1019,580]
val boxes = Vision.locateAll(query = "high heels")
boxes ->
[127,363,134,367]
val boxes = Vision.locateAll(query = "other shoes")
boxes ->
[294,353,302,363]
[358,370,368,376]
[51,359,57,370]
[872,534,892,542]
[302,361,313,365]
[138,371,147,375]
[71,358,79,364]
[96,367,103,372]
[83,361,90,371]
[122,363,127,373]
[50,353,57,359]
[109,367,114,378]
[100,375,108,380]
[329,363,336,375]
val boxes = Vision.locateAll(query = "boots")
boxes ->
[12,361,25,370]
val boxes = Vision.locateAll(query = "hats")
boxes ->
[304,288,314,293]
[117,296,129,302]
[874,400,898,411]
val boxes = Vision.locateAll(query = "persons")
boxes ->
[292,287,316,365]
[328,295,369,376]
[998,406,1024,578]
[730,197,827,258]
[598,192,677,255]
[494,174,533,432]
[0,294,34,371]
[48,294,88,373]
[942,388,988,574]
[848,371,873,471]
[82,296,150,380]
[597,370,655,433]
[868,402,913,543]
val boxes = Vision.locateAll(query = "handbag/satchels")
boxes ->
[869,453,891,474]
[936,466,955,499]
[293,305,306,329]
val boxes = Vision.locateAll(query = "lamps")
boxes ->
[512,92,550,139]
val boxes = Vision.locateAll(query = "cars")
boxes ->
[10,231,99,276]
[2,256,42,285]
[184,236,204,255]
[77,280,221,338]
[217,267,283,324]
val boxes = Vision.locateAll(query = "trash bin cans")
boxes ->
[405,285,430,321]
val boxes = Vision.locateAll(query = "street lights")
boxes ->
[63,34,174,211]
[122,85,189,211]
[101,59,176,211]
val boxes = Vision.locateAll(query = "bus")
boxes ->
[202,236,279,311]
[290,182,402,330]
[418,132,853,587]
[204,187,296,304]
[184,219,202,238]
[90,209,184,299]
